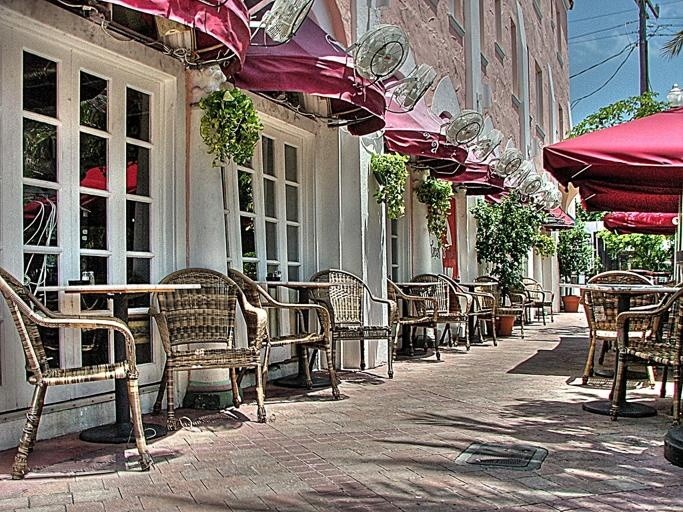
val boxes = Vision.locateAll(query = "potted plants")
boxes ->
[371,152,410,220]
[556,220,596,312]
[414,177,454,248]
[199,89,263,211]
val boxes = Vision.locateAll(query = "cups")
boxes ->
[273,271,282,282]
[83,271,94,286]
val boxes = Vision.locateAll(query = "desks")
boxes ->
[37,283,202,445]
[396,281,438,358]
[253,281,338,388]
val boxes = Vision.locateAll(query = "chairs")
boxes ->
[308,270,395,386]
[226,267,342,403]
[581,269,683,427]
[407,274,471,352]
[0,267,155,480]
[150,268,268,434]
[387,278,442,364]
[440,264,553,354]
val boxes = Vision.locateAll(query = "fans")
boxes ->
[250,0,314,47]
[442,110,564,211]
[384,63,437,113]
[346,24,410,88]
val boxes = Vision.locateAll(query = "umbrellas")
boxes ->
[543,106,682,290]
[22,156,138,221]
